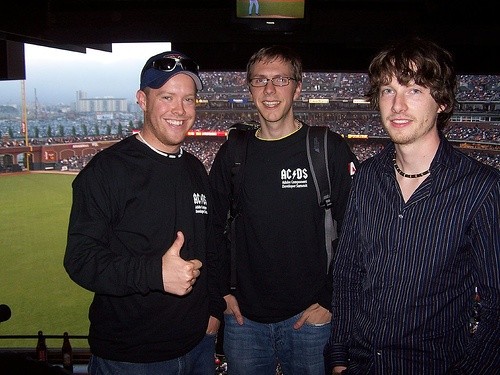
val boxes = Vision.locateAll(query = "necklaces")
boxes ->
[138,131,183,158]
[392,150,430,178]
[257,119,300,137]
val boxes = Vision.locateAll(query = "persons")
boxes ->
[470,151,500,170]
[67,154,94,169]
[299,115,385,136]
[0,141,25,147]
[181,141,223,170]
[455,75,500,102]
[300,72,370,98]
[65,51,222,375]
[442,124,500,143]
[192,112,251,131]
[209,47,359,375]
[351,143,385,163]
[198,72,248,93]
[48,135,121,144]
[331,38,500,375]
